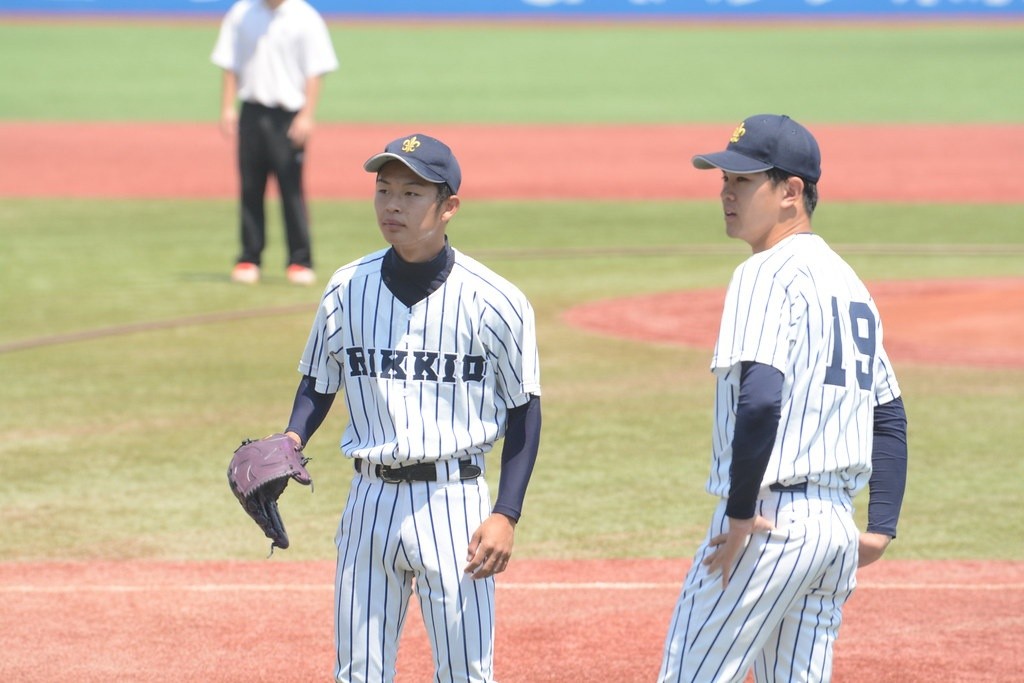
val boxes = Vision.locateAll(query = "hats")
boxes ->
[691,114,821,183]
[365,134,462,196]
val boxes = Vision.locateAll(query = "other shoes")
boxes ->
[287,265,315,285]
[233,262,259,284]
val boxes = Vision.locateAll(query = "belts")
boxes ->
[769,480,807,493]
[355,457,482,483]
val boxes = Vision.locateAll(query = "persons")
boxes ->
[212,0,339,285]
[278,129,545,683]
[657,114,908,683]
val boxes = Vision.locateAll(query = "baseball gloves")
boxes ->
[226,433,314,551]
